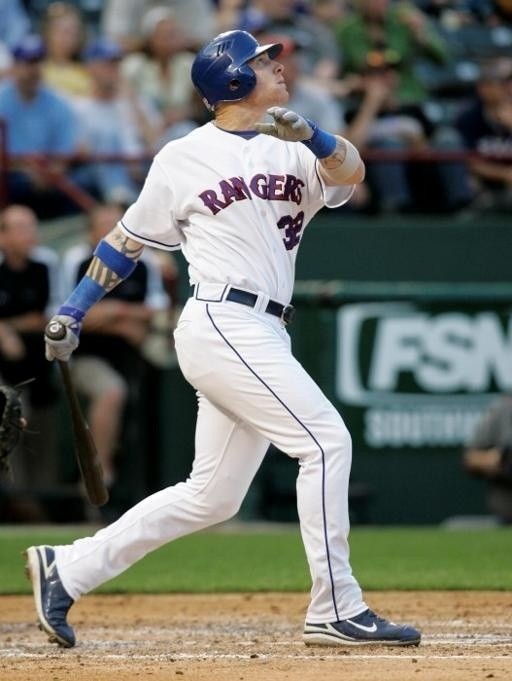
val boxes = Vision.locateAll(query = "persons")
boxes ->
[21,30,422,651]
[469,392,512,526]
[0,0,512,217]
[0,202,182,493]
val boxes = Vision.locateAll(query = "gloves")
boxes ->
[44,315,82,362]
[253,106,314,142]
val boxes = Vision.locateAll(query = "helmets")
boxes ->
[191,29,283,111]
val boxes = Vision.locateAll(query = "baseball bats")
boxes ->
[46,321,110,507]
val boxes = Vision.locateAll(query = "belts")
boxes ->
[188,284,296,325]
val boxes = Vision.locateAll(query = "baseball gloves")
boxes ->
[0,385,22,460]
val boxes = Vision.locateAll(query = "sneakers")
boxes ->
[302,608,420,647]
[21,545,75,647]
